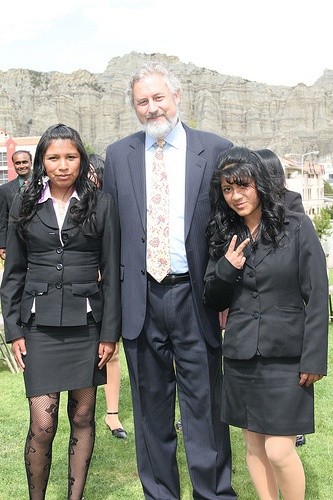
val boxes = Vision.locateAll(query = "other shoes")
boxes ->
[296,435,305,447]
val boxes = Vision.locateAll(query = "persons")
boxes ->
[101,62,238,500]
[202,146,330,500]
[0,123,129,500]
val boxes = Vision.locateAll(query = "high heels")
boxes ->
[104,412,128,439]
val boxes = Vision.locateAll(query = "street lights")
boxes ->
[302,151,320,204]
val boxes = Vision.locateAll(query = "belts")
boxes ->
[146,273,189,284]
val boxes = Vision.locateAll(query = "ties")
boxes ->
[146,141,172,283]
[24,181,29,192]
